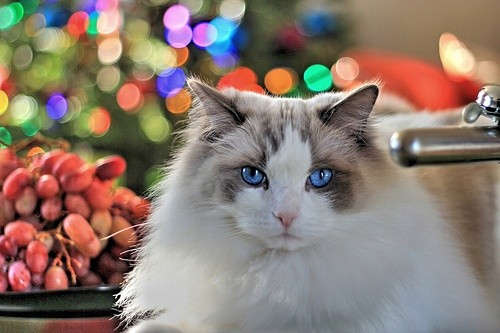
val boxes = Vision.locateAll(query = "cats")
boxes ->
[114,76,500,333]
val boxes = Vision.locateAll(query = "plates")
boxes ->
[0,285,124,315]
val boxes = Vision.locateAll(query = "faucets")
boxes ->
[388,85,500,168]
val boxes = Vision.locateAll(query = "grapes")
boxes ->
[0,146,150,292]
